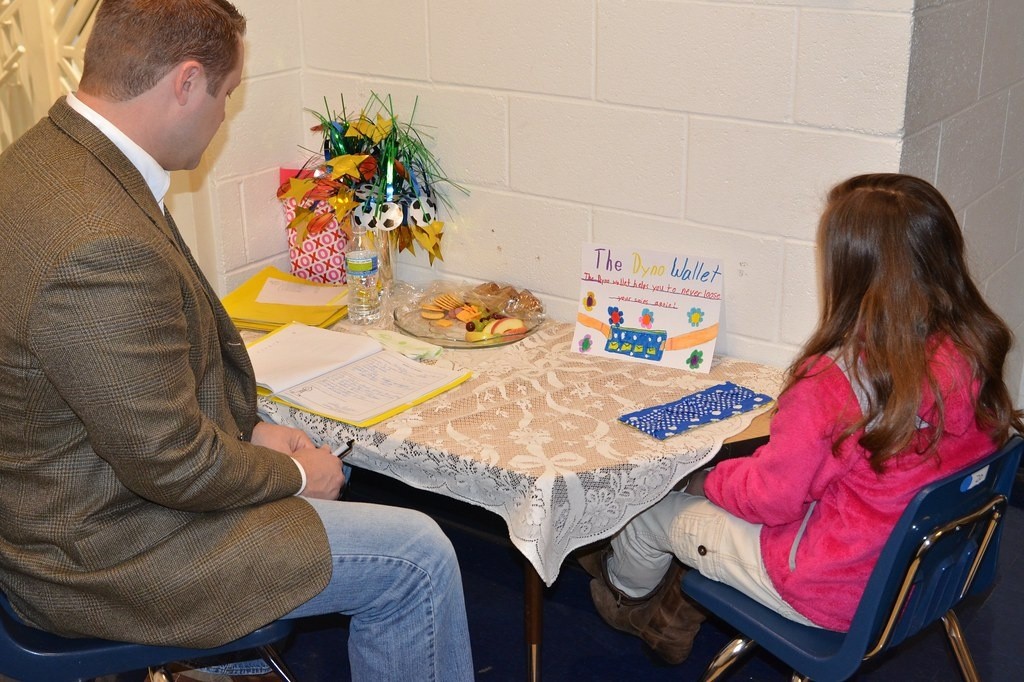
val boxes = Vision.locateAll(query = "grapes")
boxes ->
[465,302,504,333]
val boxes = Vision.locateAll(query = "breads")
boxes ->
[461,283,543,320]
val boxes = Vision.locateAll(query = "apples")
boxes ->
[466,318,528,342]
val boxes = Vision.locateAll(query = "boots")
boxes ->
[590,549,711,665]
[573,537,665,605]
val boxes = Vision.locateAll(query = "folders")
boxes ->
[219,264,380,333]
[243,320,472,430]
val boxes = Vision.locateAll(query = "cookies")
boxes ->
[421,293,482,327]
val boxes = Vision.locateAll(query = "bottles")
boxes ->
[346,226,381,325]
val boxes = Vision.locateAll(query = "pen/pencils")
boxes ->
[330,438,357,458]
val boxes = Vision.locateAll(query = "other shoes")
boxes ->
[142,658,286,682]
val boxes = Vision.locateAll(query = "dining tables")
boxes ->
[234,280,793,681]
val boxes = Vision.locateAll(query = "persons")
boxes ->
[0,2,477,681]
[574,173,1016,667]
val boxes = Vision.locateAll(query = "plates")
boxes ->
[394,297,544,348]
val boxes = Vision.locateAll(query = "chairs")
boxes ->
[678,434,1024,682]
[0,587,298,682]
